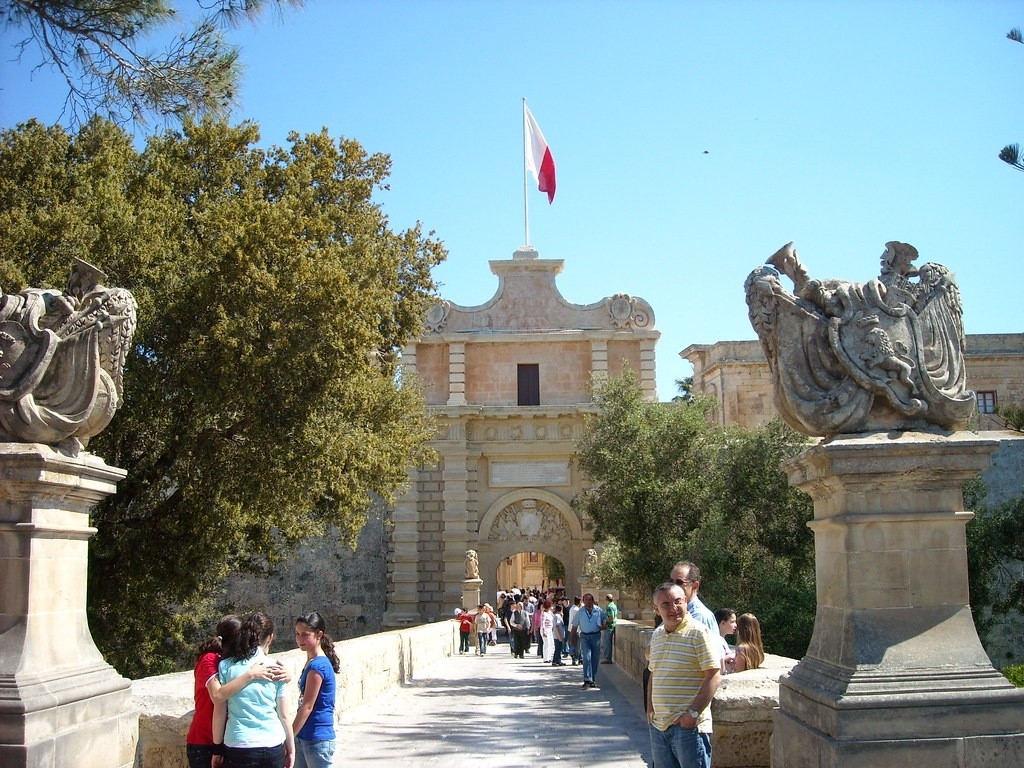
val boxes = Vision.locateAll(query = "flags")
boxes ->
[525,107,556,205]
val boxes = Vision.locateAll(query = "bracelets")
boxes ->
[212,740,224,755]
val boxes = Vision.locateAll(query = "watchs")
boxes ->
[690,709,699,719]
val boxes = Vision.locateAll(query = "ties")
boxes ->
[520,611,521,615]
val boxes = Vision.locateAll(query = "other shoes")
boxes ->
[514,652,517,658]
[552,663,559,667]
[558,661,566,665]
[480,652,484,657]
[601,660,612,664]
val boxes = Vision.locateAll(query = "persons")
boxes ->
[212,612,296,768]
[670,562,720,637]
[292,612,340,768]
[496,585,584,666]
[594,594,617,664]
[571,593,606,690]
[647,582,721,768]
[715,608,764,674]
[186,614,292,768]
[456,603,497,657]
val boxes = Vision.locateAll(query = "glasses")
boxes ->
[668,578,697,585]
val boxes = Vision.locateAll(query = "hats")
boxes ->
[559,596,570,600]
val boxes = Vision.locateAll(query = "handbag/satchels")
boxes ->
[569,646,577,655]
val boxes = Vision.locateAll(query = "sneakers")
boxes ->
[589,681,595,687]
[581,683,588,689]
